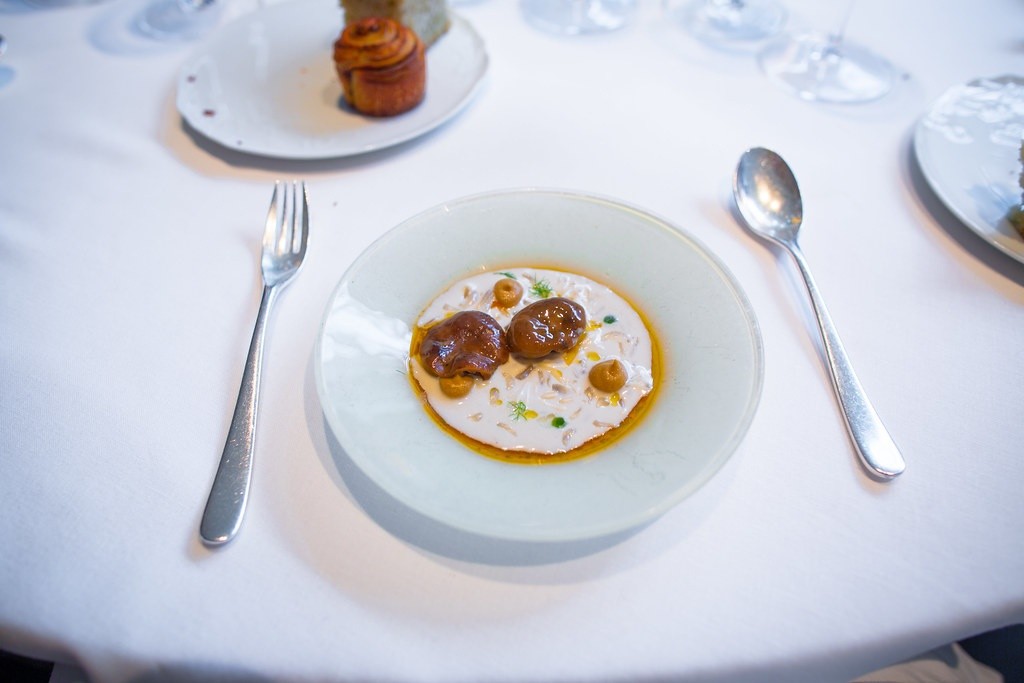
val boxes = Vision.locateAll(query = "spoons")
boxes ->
[733,146,908,479]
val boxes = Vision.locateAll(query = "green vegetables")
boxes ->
[508,401,527,422]
[533,278,552,298]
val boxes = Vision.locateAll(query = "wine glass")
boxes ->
[516,0,636,33]
[759,0,896,103]
[679,0,786,51]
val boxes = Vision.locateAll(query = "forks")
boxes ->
[199,181,308,545]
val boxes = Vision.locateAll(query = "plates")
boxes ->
[915,74,1024,263]
[178,0,491,158]
[317,189,759,545]
